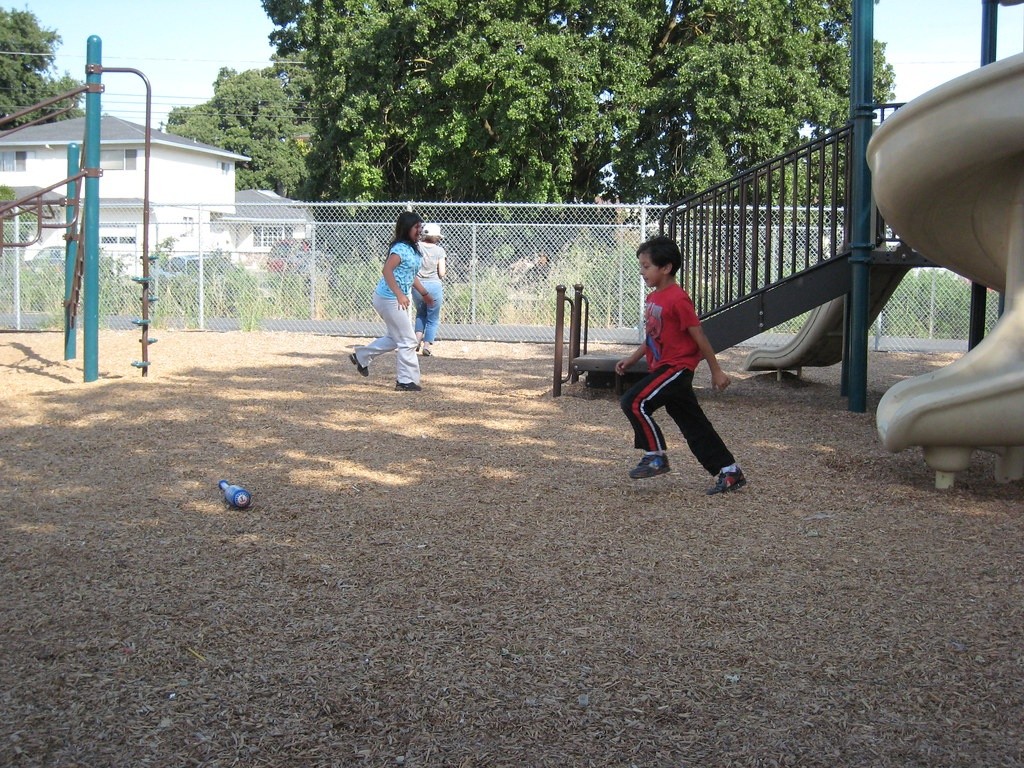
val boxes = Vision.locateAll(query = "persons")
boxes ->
[349,211,446,391]
[615,236,747,495]
[526,253,551,283]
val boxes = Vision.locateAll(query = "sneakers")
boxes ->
[630,454,670,478]
[706,467,746,496]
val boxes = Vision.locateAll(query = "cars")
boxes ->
[28,246,115,288]
[269,241,331,273]
[160,255,257,291]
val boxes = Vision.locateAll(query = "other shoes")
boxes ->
[395,381,422,391]
[348,352,369,377]
[422,348,432,356]
[416,344,420,352]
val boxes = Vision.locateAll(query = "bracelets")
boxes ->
[422,292,428,297]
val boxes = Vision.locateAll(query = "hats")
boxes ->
[422,224,445,238]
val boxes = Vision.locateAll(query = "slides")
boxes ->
[745,240,911,371]
[865,51,1024,479]
